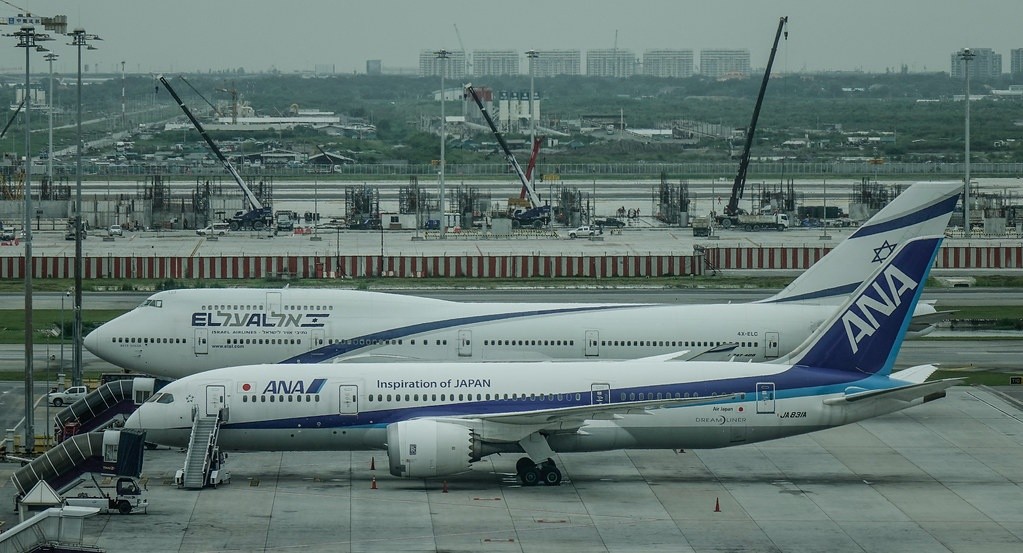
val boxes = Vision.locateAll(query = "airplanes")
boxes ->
[124,232,964,497]
[83,175,964,384]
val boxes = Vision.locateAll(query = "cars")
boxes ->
[107,223,123,237]
[2,226,16,241]
[39,124,392,180]
[794,214,859,227]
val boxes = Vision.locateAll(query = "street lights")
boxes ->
[525,48,539,192]
[2,25,59,466]
[958,44,977,238]
[42,48,60,195]
[60,26,105,389]
[434,48,454,239]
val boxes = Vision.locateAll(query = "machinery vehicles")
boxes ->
[464,83,552,230]
[155,74,275,232]
[713,13,789,228]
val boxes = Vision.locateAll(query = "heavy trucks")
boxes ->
[738,211,789,232]
[692,213,712,237]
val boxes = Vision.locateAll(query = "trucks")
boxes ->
[64,219,86,240]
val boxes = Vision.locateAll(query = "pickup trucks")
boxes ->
[568,224,601,239]
[47,385,90,407]
[592,217,627,228]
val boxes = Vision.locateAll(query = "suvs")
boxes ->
[193,223,230,236]
